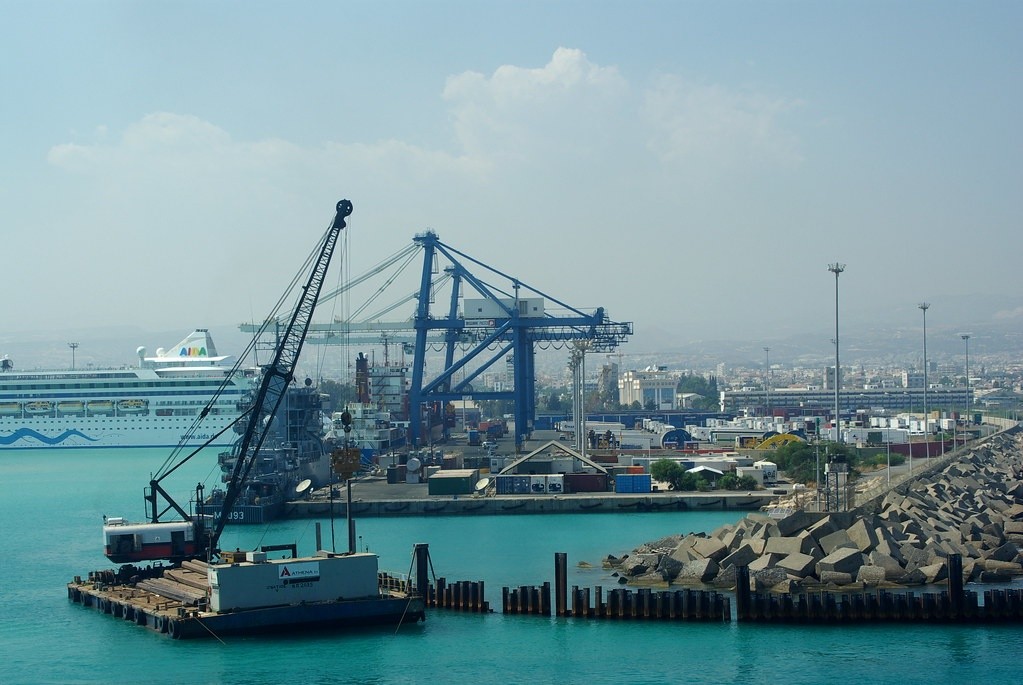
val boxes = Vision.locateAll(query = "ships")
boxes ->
[0,329,265,448]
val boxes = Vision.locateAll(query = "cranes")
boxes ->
[95,197,359,566]
[239,226,636,449]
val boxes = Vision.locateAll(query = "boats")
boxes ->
[23,399,149,416]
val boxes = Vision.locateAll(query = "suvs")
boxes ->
[481,442,498,449]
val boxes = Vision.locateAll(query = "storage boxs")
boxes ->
[428,466,652,493]
[468,421,506,442]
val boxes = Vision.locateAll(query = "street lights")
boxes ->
[960,331,971,432]
[919,301,932,460]
[66,341,79,371]
[826,263,846,440]
[763,347,771,415]
